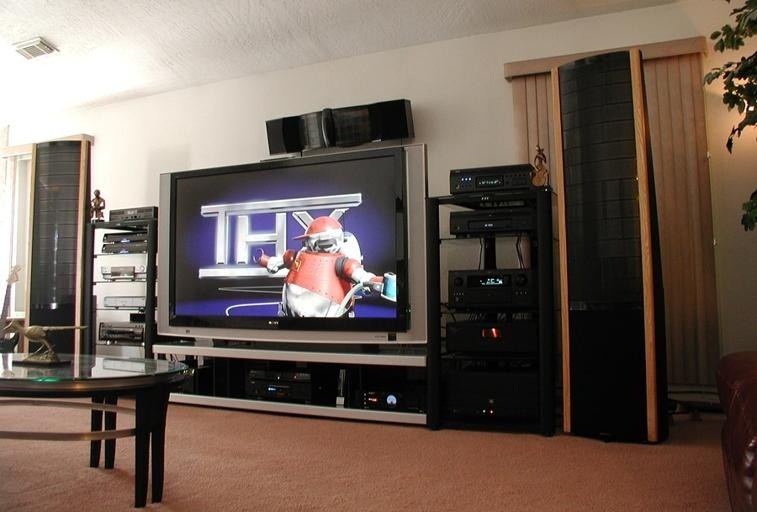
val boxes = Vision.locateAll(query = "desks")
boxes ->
[0,353,188,508]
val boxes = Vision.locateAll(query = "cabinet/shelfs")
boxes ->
[429,186,553,434]
[87,215,155,359]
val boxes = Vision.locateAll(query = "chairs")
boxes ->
[718,349,757,512]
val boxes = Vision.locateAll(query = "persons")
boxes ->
[88,190,107,219]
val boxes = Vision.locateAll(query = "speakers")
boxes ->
[320,99,415,147]
[265,111,324,156]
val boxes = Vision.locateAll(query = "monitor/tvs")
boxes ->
[157,142,427,353]
